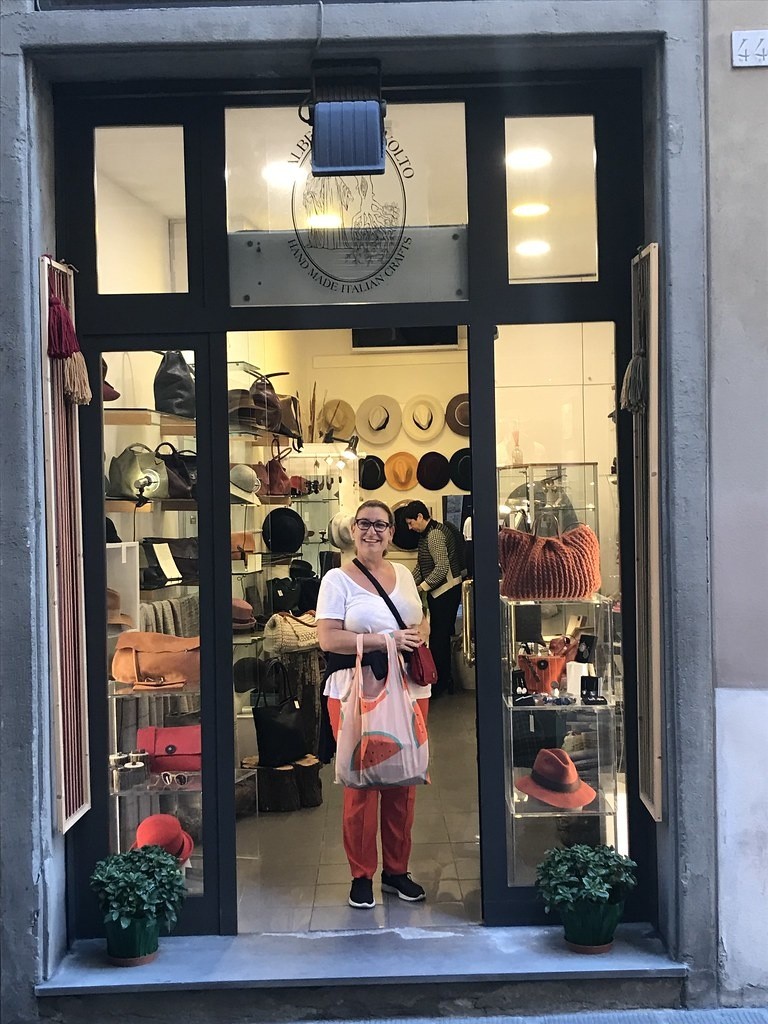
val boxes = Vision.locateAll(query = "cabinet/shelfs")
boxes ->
[100,403,296,853]
[269,441,361,577]
[496,461,603,595]
[501,584,616,890]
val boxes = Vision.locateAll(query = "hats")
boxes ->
[261,447,470,559]
[128,813,194,866]
[229,464,262,507]
[227,388,268,429]
[355,395,402,445]
[402,395,445,442]
[106,587,134,629]
[101,356,121,402]
[515,748,596,809]
[232,597,257,629]
[446,393,471,437]
[317,399,356,442]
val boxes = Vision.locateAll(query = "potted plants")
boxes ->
[534,842,637,955]
[90,841,181,968]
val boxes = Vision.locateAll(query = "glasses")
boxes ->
[354,518,391,533]
[146,771,188,793]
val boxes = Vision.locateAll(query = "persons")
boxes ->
[404,501,462,698]
[315,500,429,908]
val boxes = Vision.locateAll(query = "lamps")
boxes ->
[301,56,389,176]
[321,428,359,459]
[600,456,619,507]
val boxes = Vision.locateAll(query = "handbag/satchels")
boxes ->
[136,724,202,773]
[244,369,289,432]
[517,632,577,693]
[263,438,293,495]
[333,632,431,791]
[499,509,602,602]
[106,515,335,773]
[278,394,304,450]
[412,646,438,686]
[105,442,173,500]
[154,441,198,499]
[154,350,197,418]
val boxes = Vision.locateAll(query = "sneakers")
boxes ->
[349,876,376,908]
[380,870,426,902]
